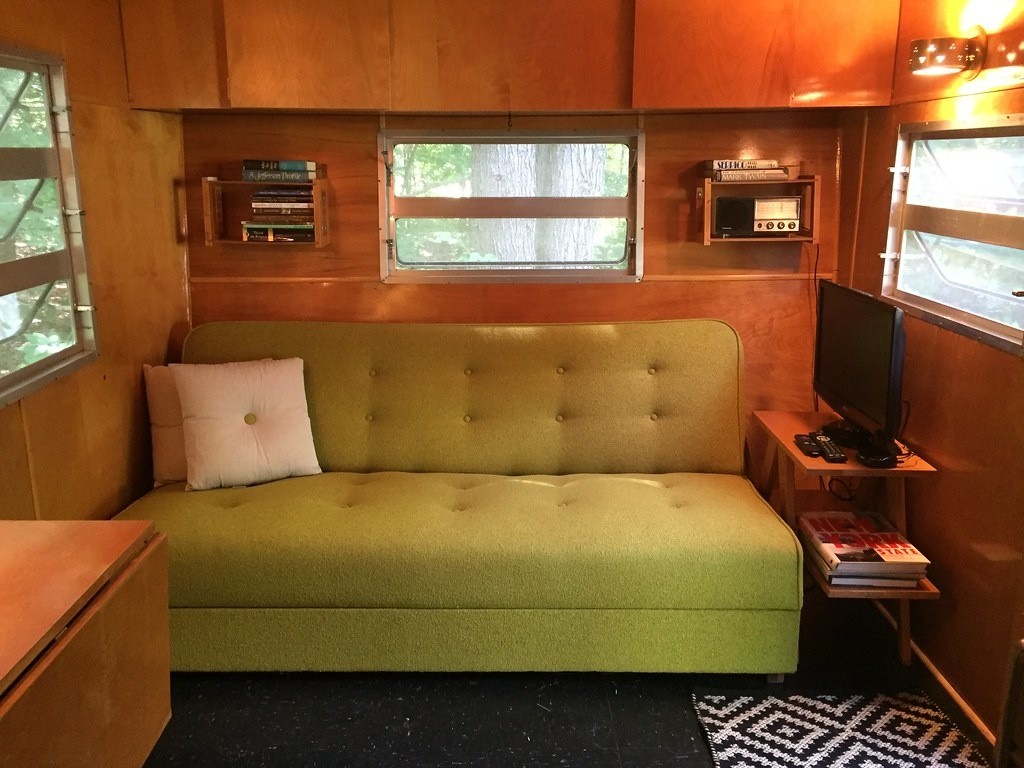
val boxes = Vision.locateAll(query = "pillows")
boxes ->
[168,356,323,492]
[143,363,187,488]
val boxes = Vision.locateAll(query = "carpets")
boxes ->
[690,690,992,768]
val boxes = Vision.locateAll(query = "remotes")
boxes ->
[809,432,848,462]
[795,434,821,456]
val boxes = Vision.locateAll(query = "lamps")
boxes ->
[906,23,987,83]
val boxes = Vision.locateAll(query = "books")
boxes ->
[704,159,780,169]
[705,167,790,182]
[241,188,315,242]
[243,159,317,171]
[799,511,931,589]
[242,171,316,182]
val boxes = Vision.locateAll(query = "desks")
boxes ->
[1,519,172,768]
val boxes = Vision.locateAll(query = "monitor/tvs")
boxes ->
[812,277,905,455]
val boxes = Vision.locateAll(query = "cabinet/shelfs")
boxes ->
[202,177,329,247]
[696,174,821,247]
[751,409,942,666]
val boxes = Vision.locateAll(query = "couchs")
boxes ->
[108,316,803,684]
